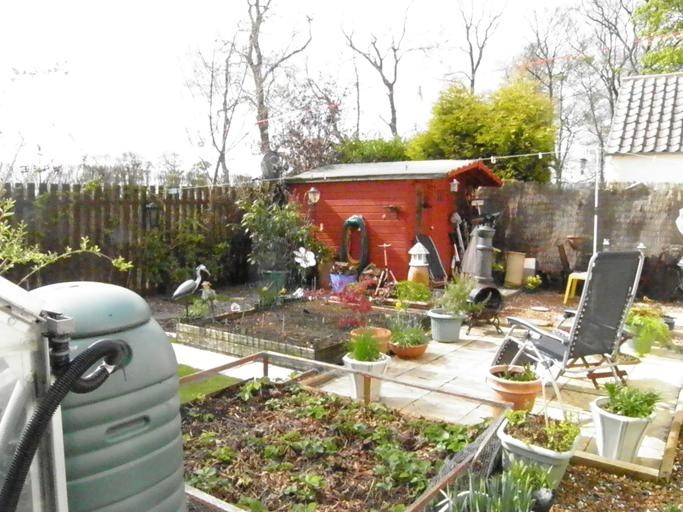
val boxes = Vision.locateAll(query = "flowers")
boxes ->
[387,302,433,351]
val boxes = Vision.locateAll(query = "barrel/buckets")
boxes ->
[329,270,357,295]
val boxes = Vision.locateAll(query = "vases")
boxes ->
[387,340,428,360]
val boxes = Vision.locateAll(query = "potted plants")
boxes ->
[337,272,391,356]
[592,378,665,464]
[624,296,675,357]
[523,274,544,293]
[234,196,337,309]
[496,410,585,490]
[342,327,391,402]
[433,455,557,512]
[329,261,358,295]
[427,272,487,343]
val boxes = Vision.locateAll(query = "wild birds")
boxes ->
[169,264,211,317]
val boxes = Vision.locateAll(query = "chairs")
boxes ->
[489,250,645,421]
[556,236,680,305]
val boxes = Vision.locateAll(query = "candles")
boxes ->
[487,364,543,418]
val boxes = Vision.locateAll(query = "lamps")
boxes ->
[307,187,320,204]
